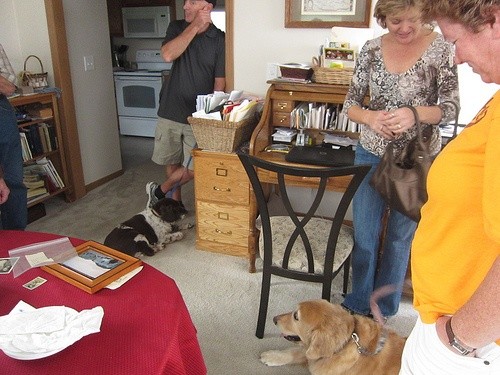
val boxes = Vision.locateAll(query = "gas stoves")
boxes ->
[114,50,174,77]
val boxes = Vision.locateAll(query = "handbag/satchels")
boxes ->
[285,143,353,166]
[22,55,49,88]
[368,100,459,222]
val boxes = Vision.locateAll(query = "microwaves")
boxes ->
[122,5,173,39]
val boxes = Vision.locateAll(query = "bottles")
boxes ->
[112,50,123,68]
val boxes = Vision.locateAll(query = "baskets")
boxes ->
[311,56,354,85]
[186,115,257,154]
[280,66,313,81]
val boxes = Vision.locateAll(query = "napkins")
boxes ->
[0,302,103,352]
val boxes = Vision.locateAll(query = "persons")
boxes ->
[145,0,226,223]
[0,43,27,230]
[400,0,500,375]
[339,0,461,328]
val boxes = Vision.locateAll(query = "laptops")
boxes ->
[285,145,356,167]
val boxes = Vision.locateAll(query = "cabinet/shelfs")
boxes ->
[249,76,370,274]
[10,89,76,207]
[190,150,257,257]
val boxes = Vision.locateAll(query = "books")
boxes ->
[19,104,64,205]
[290,102,369,133]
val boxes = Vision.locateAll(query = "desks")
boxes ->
[0,230,210,375]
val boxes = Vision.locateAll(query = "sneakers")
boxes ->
[146,181,159,208]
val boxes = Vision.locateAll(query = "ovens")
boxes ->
[114,76,164,137]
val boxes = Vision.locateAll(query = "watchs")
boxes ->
[445,317,478,357]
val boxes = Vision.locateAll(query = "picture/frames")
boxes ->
[284,0,372,28]
[40,241,140,294]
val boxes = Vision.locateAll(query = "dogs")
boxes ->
[103,197,190,258]
[260,299,407,374]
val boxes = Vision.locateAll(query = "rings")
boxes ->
[396,122,401,129]
[390,113,396,119]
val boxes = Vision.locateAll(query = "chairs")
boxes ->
[236,148,372,339]
